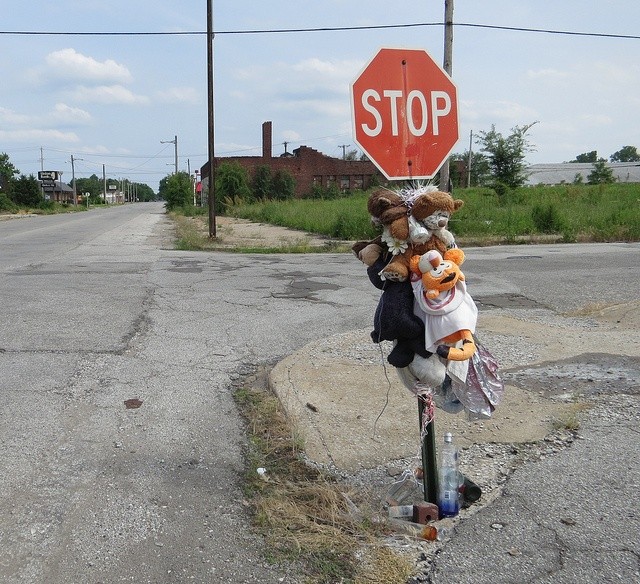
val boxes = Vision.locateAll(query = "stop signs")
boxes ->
[350,45,460,182]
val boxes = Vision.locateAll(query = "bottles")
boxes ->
[387,505,414,518]
[435,432,460,520]
[385,517,437,541]
[386,467,423,506]
[437,468,464,489]
[436,528,446,542]
[459,476,481,502]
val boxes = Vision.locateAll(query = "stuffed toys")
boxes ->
[350,184,479,396]
